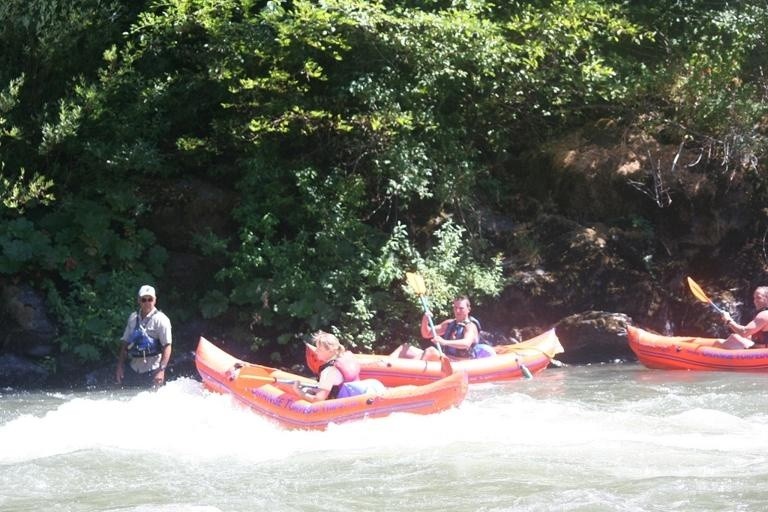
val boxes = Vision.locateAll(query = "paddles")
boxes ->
[406,272,453,376]
[687,276,725,315]
[237,367,319,389]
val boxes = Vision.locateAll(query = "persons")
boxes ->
[705,285,767,351]
[388,294,482,363]
[113,284,175,389]
[279,327,359,404]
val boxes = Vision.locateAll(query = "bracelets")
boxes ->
[724,319,732,325]
[159,365,167,370]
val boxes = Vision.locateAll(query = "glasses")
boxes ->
[141,298,153,302]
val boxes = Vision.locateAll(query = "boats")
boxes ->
[303,329,563,390]
[624,325,768,371]
[195,336,466,433]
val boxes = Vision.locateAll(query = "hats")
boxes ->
[138,285,155,297]
